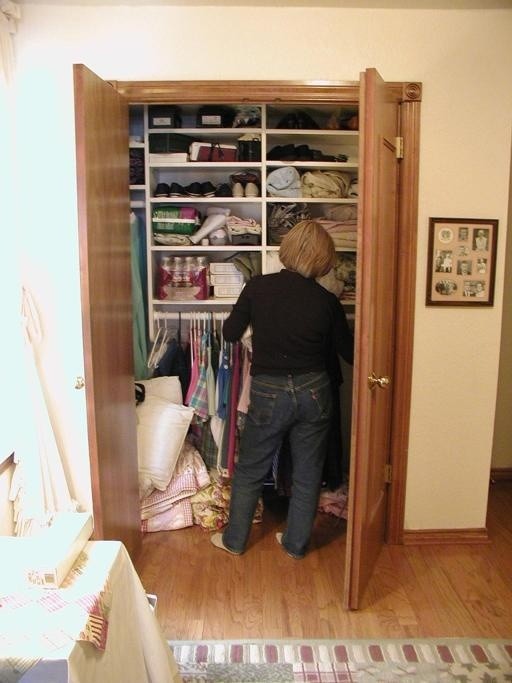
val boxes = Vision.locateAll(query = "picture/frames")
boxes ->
[425,217,499,307]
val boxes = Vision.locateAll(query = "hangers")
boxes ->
[147,312,232,368]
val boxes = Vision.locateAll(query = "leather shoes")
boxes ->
[276,110,358,130]
[266,144,335,161]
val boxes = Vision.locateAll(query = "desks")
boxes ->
[2,533,181,683]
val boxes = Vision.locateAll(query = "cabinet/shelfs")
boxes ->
[128,103,358,304]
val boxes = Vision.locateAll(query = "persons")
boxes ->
[435,226,488,298]
[211,220,351,560]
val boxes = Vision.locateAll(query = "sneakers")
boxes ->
[154,171,259,198]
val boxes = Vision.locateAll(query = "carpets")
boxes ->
[166,637,512,683]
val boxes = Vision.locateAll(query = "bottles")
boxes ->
[157,255,210,299]
